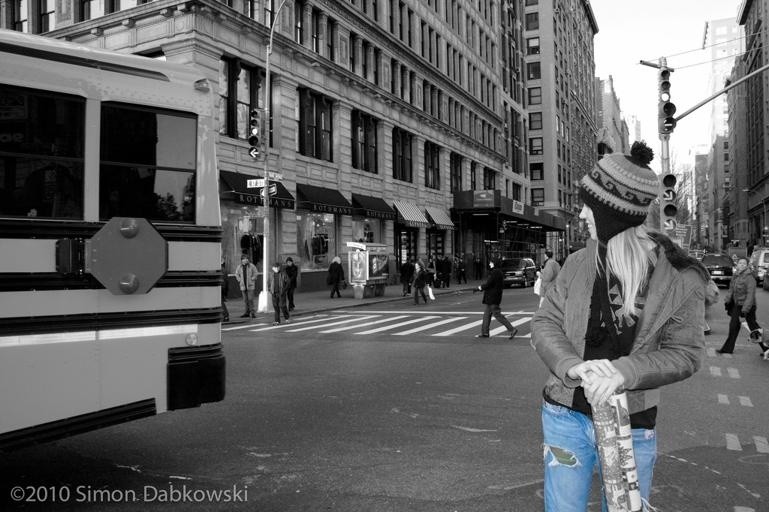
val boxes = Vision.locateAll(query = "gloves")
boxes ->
[473,287,479,294]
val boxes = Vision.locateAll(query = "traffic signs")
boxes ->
[259,183,278,199]
[246,178,266,189]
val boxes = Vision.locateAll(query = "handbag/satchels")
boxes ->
[534,276,542,295]
[725,293,735,317]
[411,286,421,297]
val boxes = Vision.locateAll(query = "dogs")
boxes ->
[748,327,769,360]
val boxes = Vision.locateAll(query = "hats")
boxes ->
[578,141,660,239]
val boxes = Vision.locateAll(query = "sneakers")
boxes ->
[273,320,290,326]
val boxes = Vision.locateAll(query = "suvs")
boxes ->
[687,250,705,263]
[501,257,537,287]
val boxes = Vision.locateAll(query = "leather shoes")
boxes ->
[509,329,517,340]
[479,334,489,337]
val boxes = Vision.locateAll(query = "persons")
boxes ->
[530,141,710,512]
[220,248,768,360]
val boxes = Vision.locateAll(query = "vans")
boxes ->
[747,247,769,286]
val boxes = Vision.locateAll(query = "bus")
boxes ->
[1,30,225,460]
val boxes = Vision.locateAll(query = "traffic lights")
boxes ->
[248,105,261,161]
[662,173,679,235]
[659,67,677,135]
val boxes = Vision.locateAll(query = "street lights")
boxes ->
[742,187,767,246]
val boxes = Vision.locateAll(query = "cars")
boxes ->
[702,253,734,288]
